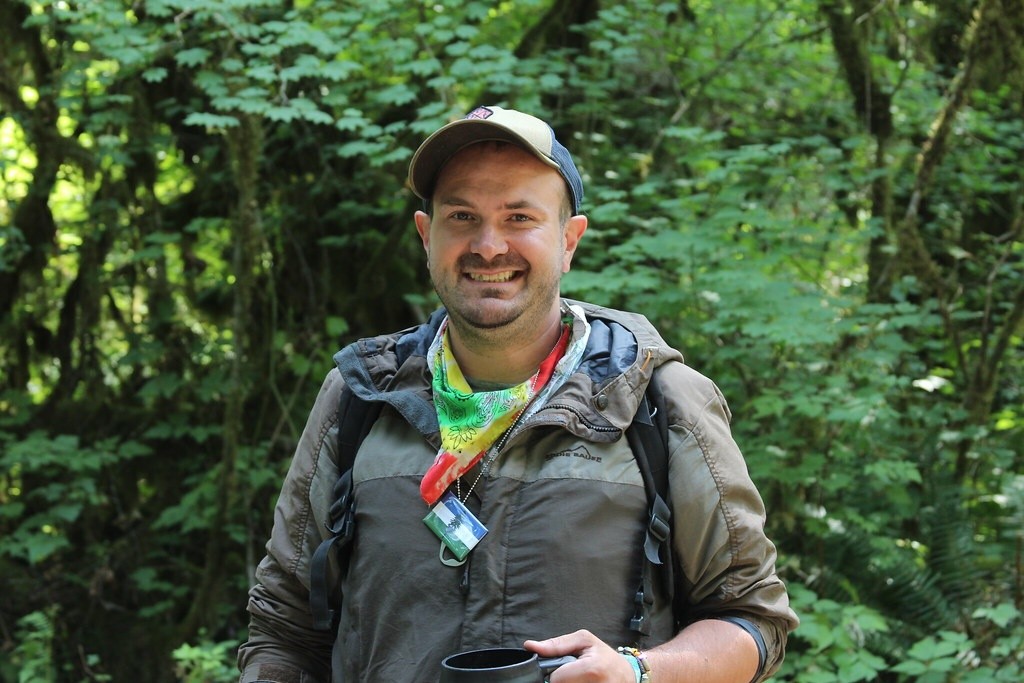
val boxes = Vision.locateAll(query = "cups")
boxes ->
[438,648,577,683]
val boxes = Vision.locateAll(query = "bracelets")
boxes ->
[616,645,651,683]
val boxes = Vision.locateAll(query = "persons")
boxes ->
[230,104,799,683]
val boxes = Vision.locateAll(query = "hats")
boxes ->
[409,106,584,216]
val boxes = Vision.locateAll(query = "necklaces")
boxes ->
[438,332,563,567]
